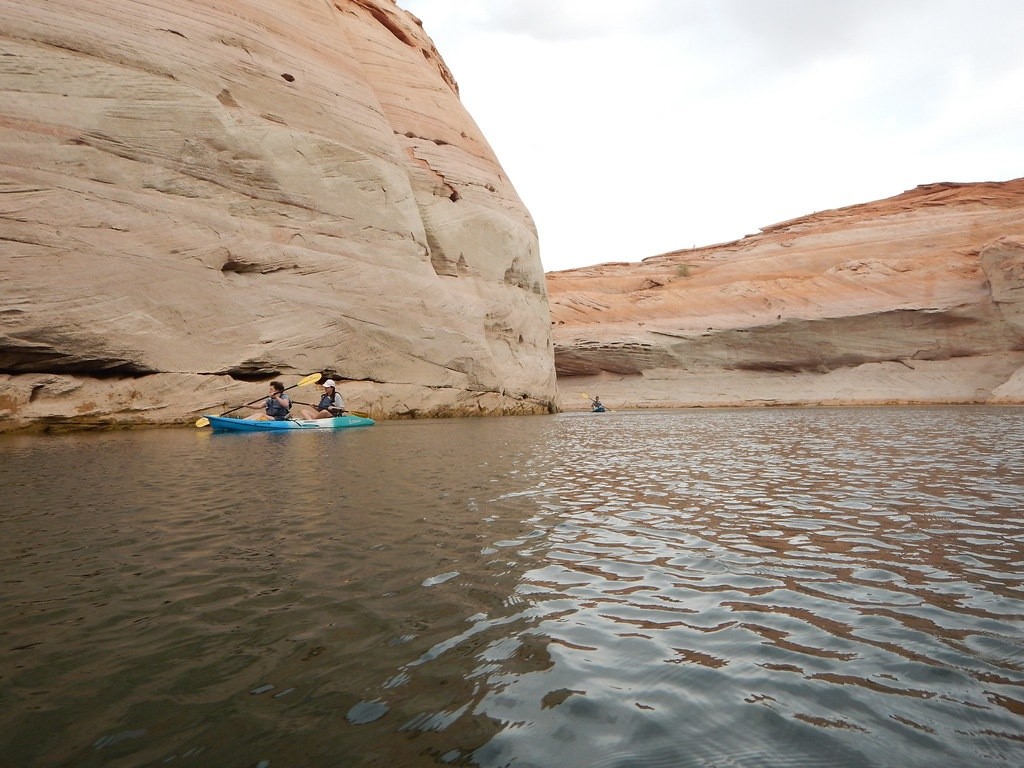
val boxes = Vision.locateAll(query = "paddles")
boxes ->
[581,391,616,412]
[291,399,369,419]
[194,371,322,428]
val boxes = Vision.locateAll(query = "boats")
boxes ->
[203,415,376,432]
[590,406,605,412]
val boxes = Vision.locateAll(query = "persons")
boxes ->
[242,381,292,421]
[302,380,344,419]
[591,396,603,409]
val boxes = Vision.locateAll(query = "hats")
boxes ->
[323,379,335,388]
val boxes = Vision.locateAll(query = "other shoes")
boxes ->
[226,415,230,418]
[236,415,243,419]
